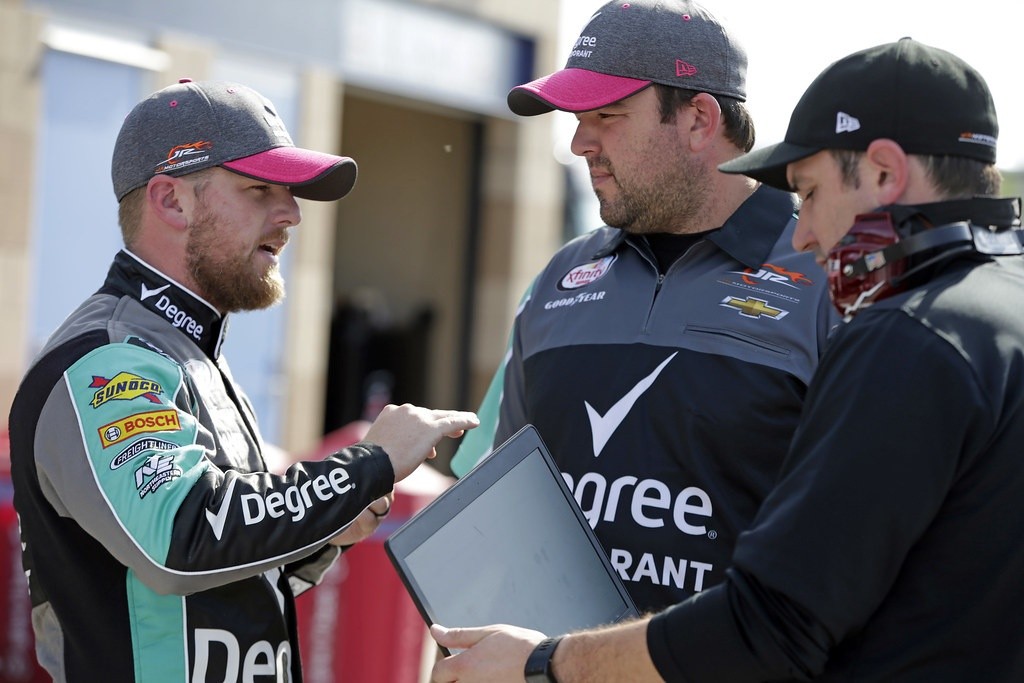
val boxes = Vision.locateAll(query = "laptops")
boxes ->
[384,423,642,660]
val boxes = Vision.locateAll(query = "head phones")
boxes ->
[826,194,1024,324]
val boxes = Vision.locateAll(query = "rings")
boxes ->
[368,502,390,517]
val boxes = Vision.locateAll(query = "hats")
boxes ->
[112,78,359,204]
[718,37,999,192]
[507,0,749,117]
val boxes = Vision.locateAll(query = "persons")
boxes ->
[430,0,1024,683]
[8,77,480,683]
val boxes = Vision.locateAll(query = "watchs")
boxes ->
[524,636,564,683]
